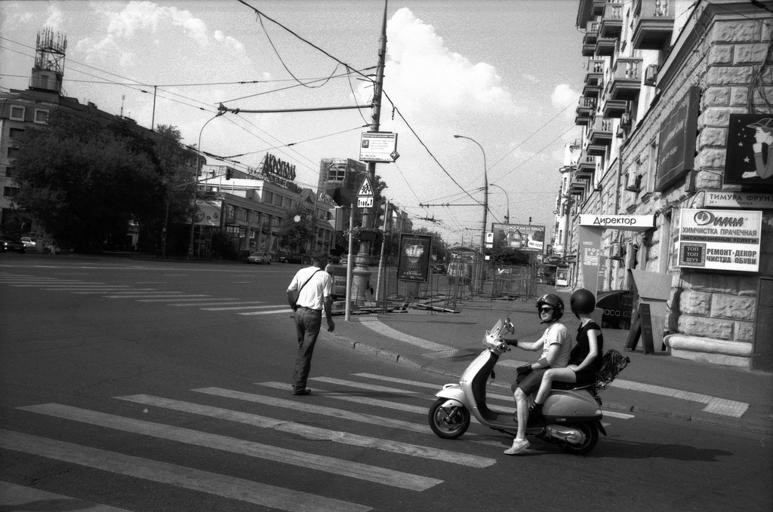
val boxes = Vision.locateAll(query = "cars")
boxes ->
[0,233,40,253]
[246,251,272,264]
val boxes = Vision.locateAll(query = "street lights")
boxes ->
[453,133,516,297]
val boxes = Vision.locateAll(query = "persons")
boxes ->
[499,292,573,457]
[285,250,335,397]
[511,288,604,426]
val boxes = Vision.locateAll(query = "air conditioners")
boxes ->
[612,112,631,137]
[643,65,658,84]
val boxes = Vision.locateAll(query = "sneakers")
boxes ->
[504,438,531,455]
[292,385,311,395]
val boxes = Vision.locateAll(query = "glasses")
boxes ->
[538,307,553,313]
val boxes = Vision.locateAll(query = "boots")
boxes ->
[514,400,542,425]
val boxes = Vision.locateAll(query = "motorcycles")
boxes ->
[427,316,630,455]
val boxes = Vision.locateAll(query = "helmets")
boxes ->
[570,288,595,313]
[536,294,564,319]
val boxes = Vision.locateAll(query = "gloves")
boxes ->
[517,365,532,375]
[499,339,517,347]
